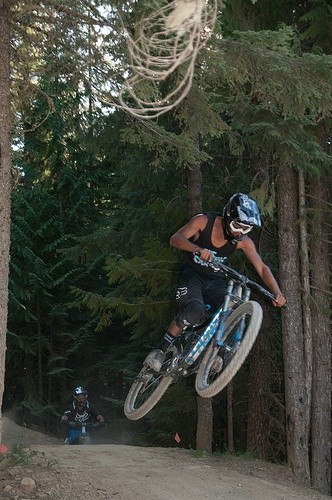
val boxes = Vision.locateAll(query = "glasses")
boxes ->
[230,220,253,234]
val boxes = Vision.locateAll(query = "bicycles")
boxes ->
[122,249,286,420]
[68,420,108,446]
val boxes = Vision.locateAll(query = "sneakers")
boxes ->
[143,349,166,372]
[196,356,223,377]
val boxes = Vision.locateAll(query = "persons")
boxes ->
[60,385,105,444]
[142,191,286,373]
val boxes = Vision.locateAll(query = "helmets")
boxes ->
[73,386,88,403]
[221,193,262,244]
